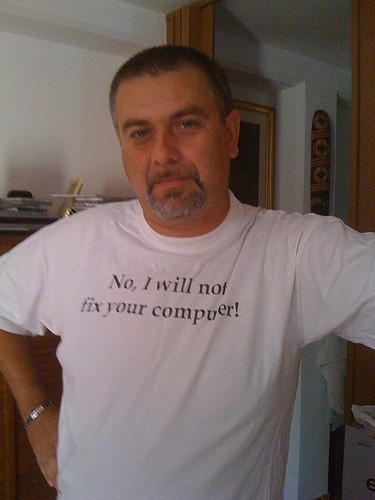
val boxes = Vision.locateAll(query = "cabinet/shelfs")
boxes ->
[0,230,62,500]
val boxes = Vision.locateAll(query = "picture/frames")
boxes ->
[226,98,274,211]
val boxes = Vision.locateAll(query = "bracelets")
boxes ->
[24,397,55,430]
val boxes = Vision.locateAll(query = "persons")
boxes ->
[0,39,374,500]
